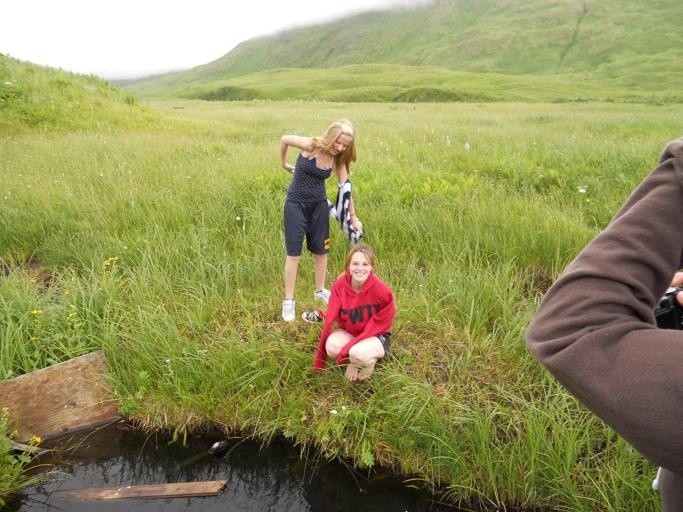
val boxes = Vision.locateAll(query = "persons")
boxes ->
[312,242,396,382]
[523,135,683,512]
[279,119,363,323]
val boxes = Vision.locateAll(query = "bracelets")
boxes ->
[350,213,355,216]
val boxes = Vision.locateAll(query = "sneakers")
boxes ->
[302,311,324,323]
[282,298,296,322]
[313,289,331,304]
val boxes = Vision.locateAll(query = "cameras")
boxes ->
[651,282,683,330]
